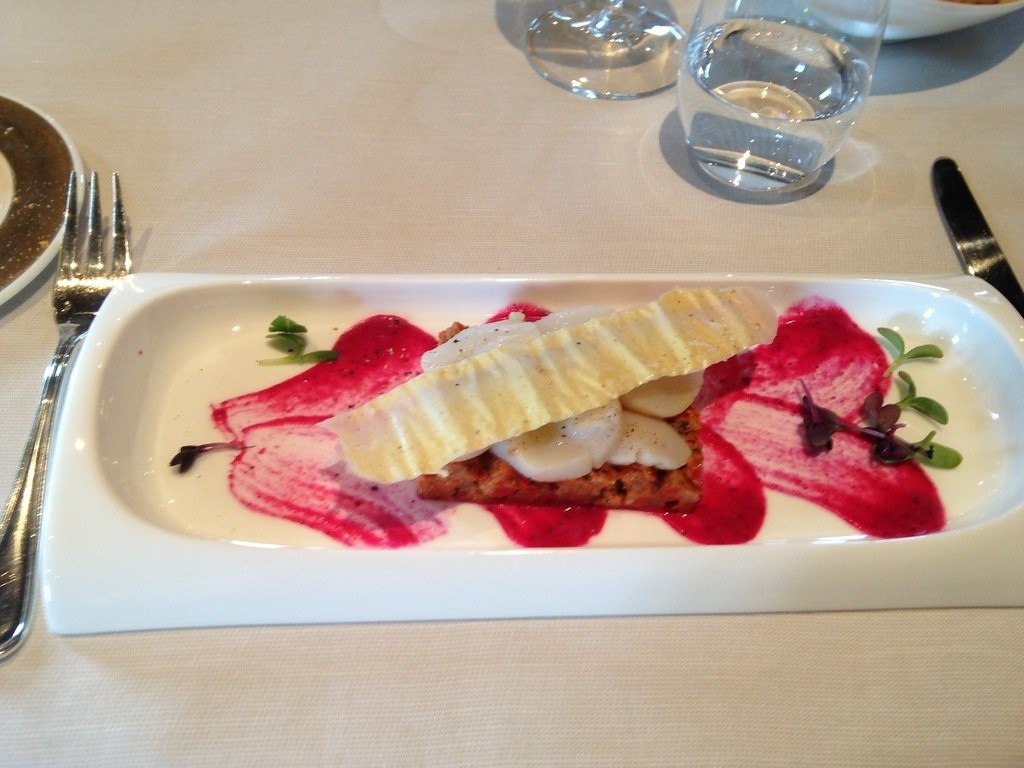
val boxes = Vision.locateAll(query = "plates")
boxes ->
[0,94,87,312]
[37,272,1024,619]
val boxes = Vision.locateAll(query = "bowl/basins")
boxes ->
[797,0,1024,40]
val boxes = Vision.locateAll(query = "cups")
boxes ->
[675,0,892,192]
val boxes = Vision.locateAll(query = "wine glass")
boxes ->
[524,1,690,100]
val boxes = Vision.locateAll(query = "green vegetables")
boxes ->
[875,327,964,470]
[259,315,338,367]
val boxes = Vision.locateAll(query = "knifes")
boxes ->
[931,155,1024,327]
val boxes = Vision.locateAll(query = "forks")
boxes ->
[0,167,130,664]
